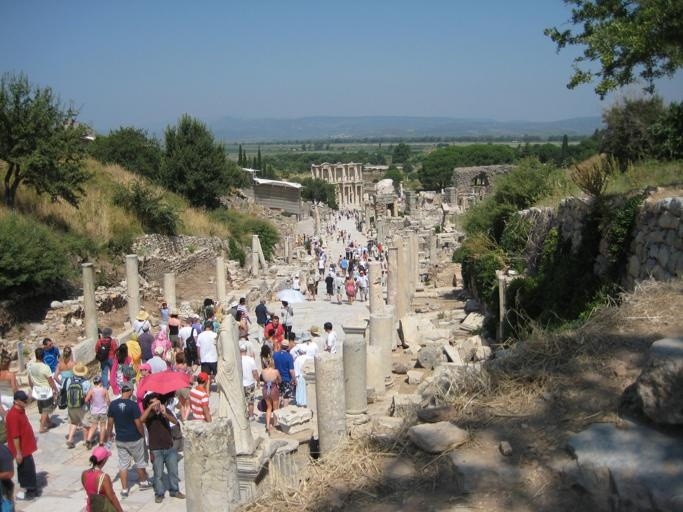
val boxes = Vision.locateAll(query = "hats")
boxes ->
[142,323,149,331]
[92,447,112,463]
[103,328,113,337]
[301,331,311,341]
[138,363,152,372]
[141,390,167,409]
[306,326,321,335]
[239,344,247,351]
[93,376,100,384]
[121,382,134,391]
[198,372,208,383]
[135,310,150,321]
[72,363,88,376]
[154,346,164,355]
[281,339,289,347]
[14,391,28,400]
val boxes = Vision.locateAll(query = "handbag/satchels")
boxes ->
[32,385,54,401]
[89,471,119,512]
[58,378,68,409]
[124,366,137,378]
[165,347,176,365]
[258,399,273,412]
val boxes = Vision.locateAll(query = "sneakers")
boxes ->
[155,493,164,504]
[15,488,42,500]
[169,490,185,500]
[139,480,154,490]
[65,441,75,448]
[86,439,96,450]
[120,486,129,497]
[47,423,57,430]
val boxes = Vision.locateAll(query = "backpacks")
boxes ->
[97,336,112,363]
[186,328,197,361]
[68,376,88,409]
